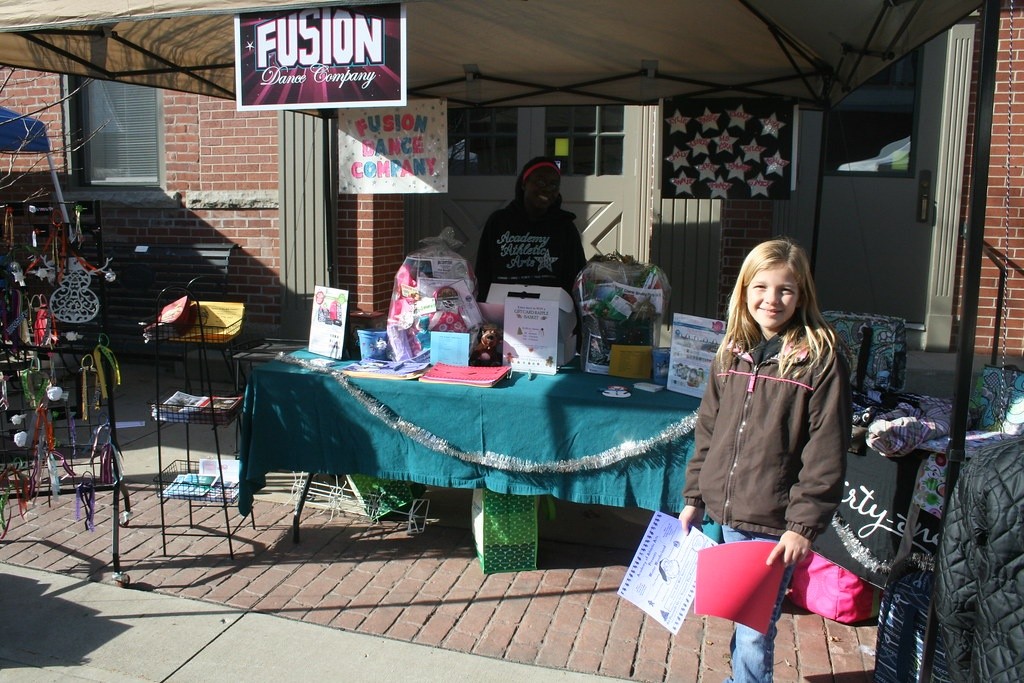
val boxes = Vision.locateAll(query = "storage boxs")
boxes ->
[486,282,578,367]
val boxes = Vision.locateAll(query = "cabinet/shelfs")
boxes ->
[139,274,256,562]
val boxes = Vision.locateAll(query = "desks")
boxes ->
[238,331,722,546]
[225,336,346,459]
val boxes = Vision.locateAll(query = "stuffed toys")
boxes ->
[470,324,504,366]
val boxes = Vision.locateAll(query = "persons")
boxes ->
[474,157,590,354]
[679,238,854,683]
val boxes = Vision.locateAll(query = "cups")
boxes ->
[357,328,387,361]
[652,348,670,385]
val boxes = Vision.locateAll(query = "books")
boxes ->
[153,390,243,425]
[157,458,240,503]
[342,354,429,380]
[419,360,511,387]
[142,295,198,342]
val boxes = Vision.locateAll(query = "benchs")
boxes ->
[19,242,239,384]
